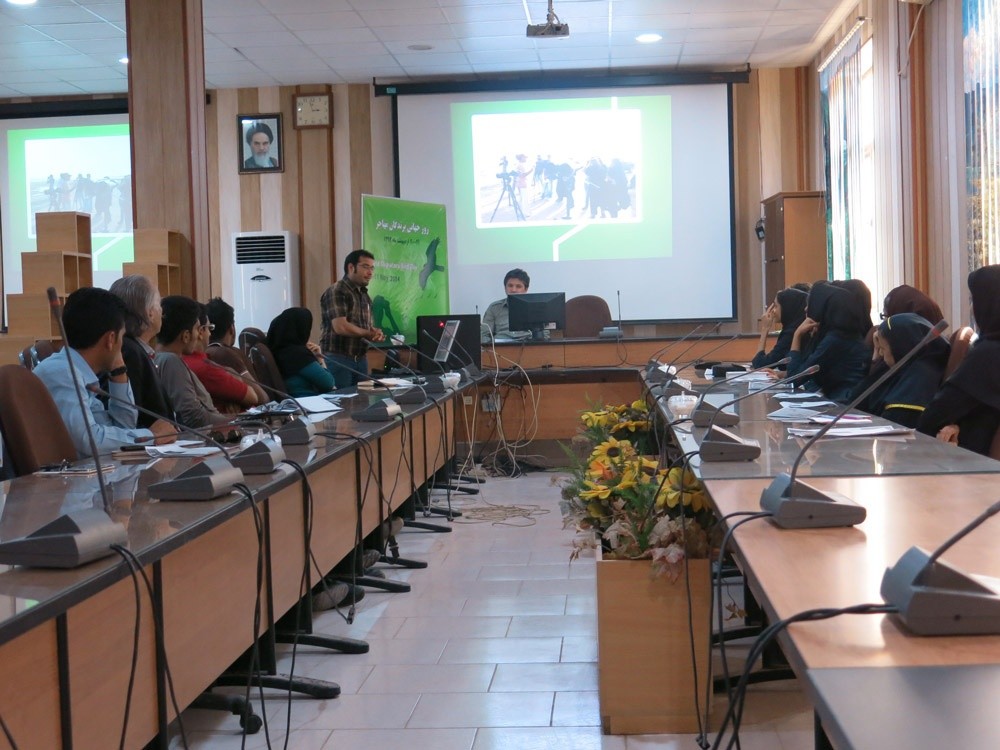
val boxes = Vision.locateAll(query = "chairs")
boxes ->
[19,340,53,369]
[566,295,612,339]
[239,328,288,402]
[0,363,78,475]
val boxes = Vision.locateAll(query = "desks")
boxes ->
[701,472,1000,687]
[800,663,1000,750]
[0,372,489,750]
[372,330,782,445]
[640,366,1000,644]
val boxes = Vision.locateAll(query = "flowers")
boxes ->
[547,390,713,585]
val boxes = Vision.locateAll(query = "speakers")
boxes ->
[416,314,481,370]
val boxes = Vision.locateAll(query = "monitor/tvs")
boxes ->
[507,293,567,340]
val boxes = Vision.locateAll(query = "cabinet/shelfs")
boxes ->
[760,192,827,310]
[0,211,94,366]
[121,228,182,348]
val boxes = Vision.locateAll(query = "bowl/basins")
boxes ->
[240,429,281,450]
[672,378,691,391]
[658,363,676,375]
[442,370,461,388]
[668,391,697,417]
[390,333,404,345]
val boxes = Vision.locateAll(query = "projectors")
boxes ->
[528,22,571,39]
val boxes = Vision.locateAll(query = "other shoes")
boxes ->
[312,583,349,611]
[362,549,381,568]
[365,569,386,580]
[334,586,364,608]
[384,518,404,536]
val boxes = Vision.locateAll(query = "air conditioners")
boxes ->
[232,231,301,347]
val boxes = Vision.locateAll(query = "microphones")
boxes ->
[662,333,743,401]
[0,286,132,564]
[420,322,480,383]
[389,335,460,389]
[649,322,724,384]
[690,355,791,429]
[363,338,435,397]
[316,352,402,421]
[644,325,703,380]
[698,363,819,461]
[133,420,289,473]
[86,383,244,499]
[880,502,1000,636]
[204,358,317,443]
[758,319,950,529]
[597,290,623,338]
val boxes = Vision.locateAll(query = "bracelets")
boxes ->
[107,365,128,378]
[319,361,325,365]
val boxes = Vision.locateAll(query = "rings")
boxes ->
[937,430,942,433]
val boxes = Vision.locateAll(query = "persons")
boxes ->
[31,275,271,457]
[481,267,551,346]
[500,154,637,220]
[751,263,1000,456]
[267,306,335,398]
[319,249,387,389]
[241,120,278,169]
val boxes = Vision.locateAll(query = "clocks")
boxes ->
[291,90,334,129]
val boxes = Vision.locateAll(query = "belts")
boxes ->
[324,351,367,362]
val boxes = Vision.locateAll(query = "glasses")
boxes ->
[199,325,215,331]
[880,313,890,320]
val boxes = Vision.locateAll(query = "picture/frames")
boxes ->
[236,112,285,175]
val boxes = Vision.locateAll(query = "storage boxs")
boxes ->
[591,528,713,735]
